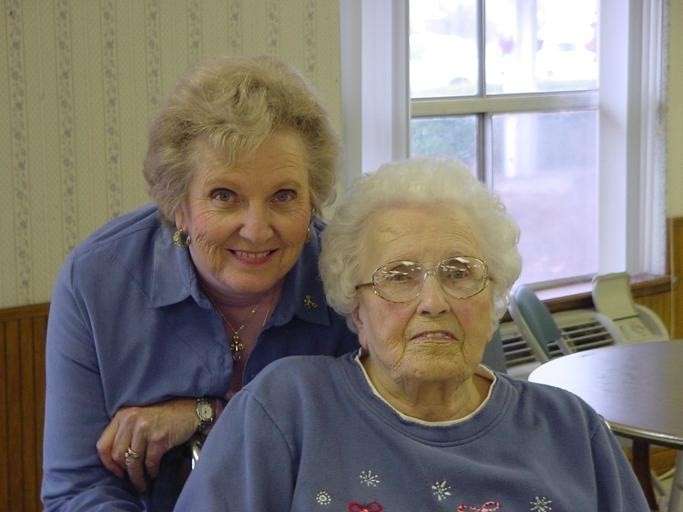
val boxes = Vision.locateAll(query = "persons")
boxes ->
[41,58,361,512]
[175,157,651,512]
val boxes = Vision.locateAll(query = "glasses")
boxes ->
[355,256,494,303]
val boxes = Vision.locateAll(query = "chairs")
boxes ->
[507,273,670,493]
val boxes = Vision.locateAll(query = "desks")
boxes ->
[527,336,683,511]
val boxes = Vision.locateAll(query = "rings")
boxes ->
[127,447,140,459]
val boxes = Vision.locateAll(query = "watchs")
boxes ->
[196,397,215,434]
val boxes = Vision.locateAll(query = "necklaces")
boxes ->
[205,288,276,361]
